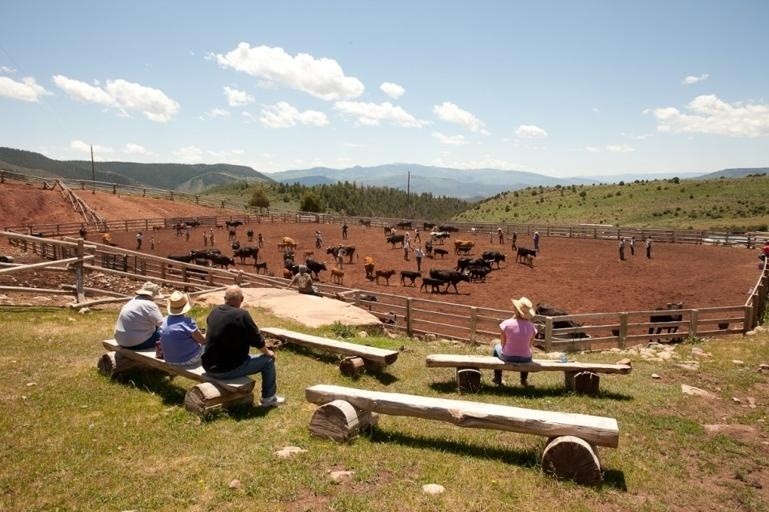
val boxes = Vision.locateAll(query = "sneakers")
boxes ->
[492,378,501,384]
[521,379,526,385]
[259,395,285,407]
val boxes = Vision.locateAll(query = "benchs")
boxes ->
[97,340,256,417]
[424,353,633,395]
[260,327,398,375]
[304,384,619,481]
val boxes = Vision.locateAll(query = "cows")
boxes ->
[80,223,87,240]
[153,226,161,233]
[103,232,112,244]
[648,301,684,342]
[516,248,536,266]
[168,228,355,287]
[359,219,371,227]
[376,222,505,293]
[364,255,375,278]
[535,302,589,339]
[226,220,243,230]
[172,221,200,230]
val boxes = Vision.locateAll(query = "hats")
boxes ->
[135,281,165,299]
[167,291,192,315]
[511,296,535,319]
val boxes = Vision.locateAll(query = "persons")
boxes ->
[135,231,143,250]
[113,281,163,350]
[511,230,518,251]
[335,244,345,270]
[341,222,349,239]
[746,234,769,261]
[156,290,207,367]
[201,286,285,408]
[618,236,653,260]
[176,220,264,247]
[315,229,323,248]
[287,264,321,296]
[151,235,155,250]
[532,232,539,250]
[390,224,439,271]
[497,228,504,244]
[491,297,538,384]
